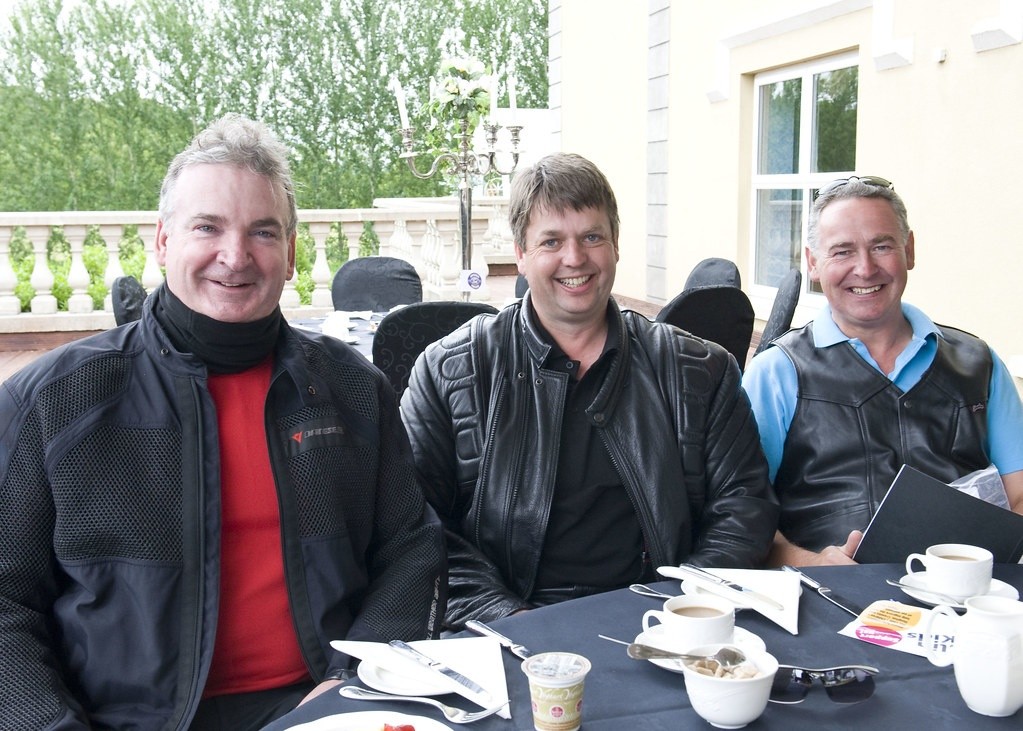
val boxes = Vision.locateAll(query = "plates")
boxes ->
[633,625,766,673]
[899,573,1019,610]
[357,646,478,695]
[284,711,453,731]
[679,580,803,610]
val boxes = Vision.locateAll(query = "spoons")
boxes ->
[626,642,747,671]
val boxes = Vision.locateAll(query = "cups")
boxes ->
[642,594,736,654]
[319,315,350,342]
[923,597,1023,716]
[906,543,994,600]
[521,653,592,731]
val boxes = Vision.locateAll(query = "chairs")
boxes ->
[371,301,500,400]
[655,284,756,376]
[754,267,803,359]
[332,256,423,313]
[682,257,742,290]
[111,276,148,327]
[515,273,530,298]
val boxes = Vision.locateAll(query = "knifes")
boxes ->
[465,619,534,660]
[390,639,494,706]
[783,564,862,617]
[683,564,782,611]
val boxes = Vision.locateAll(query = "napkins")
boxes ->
[320,310,372,341]
[329,637,513,722]
[656,564,801,637]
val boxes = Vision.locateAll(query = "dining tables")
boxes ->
[257,562,1023,731]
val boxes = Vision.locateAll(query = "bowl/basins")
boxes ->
[682,645,780,729]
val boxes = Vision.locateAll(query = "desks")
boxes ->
[287,313,389,364]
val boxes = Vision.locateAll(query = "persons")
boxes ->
[399,151,774,632]
[0,111,444,731]
[735,176,1023,565]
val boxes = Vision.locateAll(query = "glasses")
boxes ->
[813,176,895,201]
[768,663,881,706]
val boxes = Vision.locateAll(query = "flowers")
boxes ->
[417,55,492,129]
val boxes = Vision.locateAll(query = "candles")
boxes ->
[508,74,519,125]
[428,75,438,125]
[392,77,410,129]
[490,72,497,125]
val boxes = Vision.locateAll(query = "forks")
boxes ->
[339,686,504,724]
